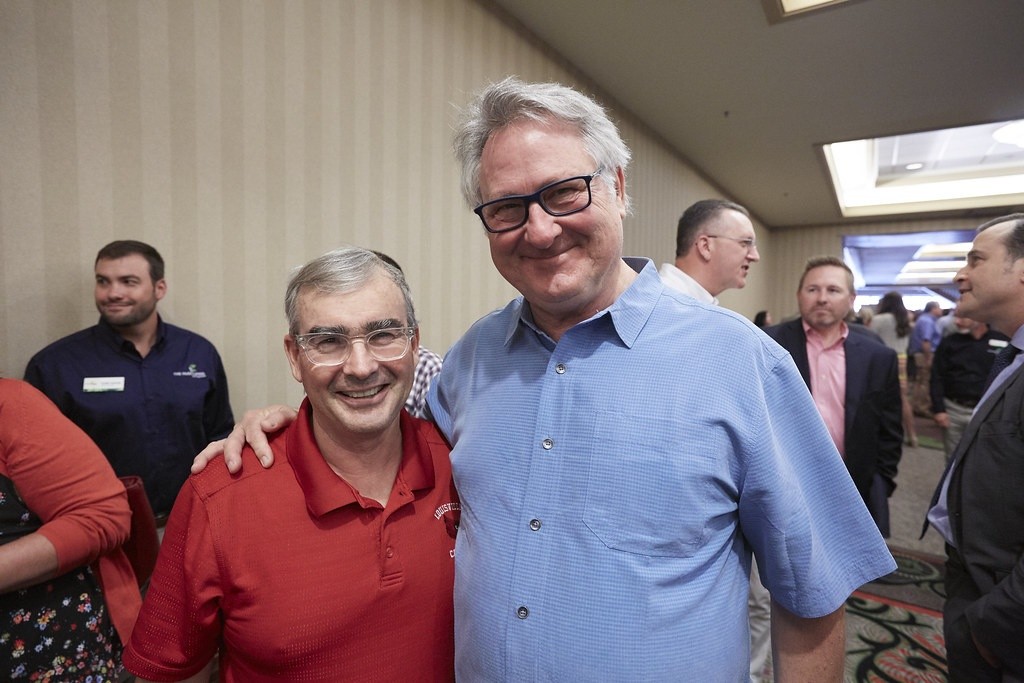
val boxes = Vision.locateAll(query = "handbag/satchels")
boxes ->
[90,477,161,591]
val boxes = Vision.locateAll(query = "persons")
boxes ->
[910,301,956,418]
[846,307,871,325]
[929,318,1012,462]
[117,246,461,683]
[868,292,918,447]
[373,251,444,419]
[660,199,759,305]
[761,256,904,538]
[191,78,898,683]
[754,311,771,328]
[23,239,235,544]
[0,377,142,683]
[919,213,1024,683]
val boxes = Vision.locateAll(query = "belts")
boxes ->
[154,514,168,528]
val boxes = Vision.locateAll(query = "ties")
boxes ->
[917,344,1022,540]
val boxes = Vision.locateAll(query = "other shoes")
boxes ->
[908,437,917,447]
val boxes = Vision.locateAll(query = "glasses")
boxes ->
[474,166,605,233]
[295,327,415,366]
[695,235,757,249]
